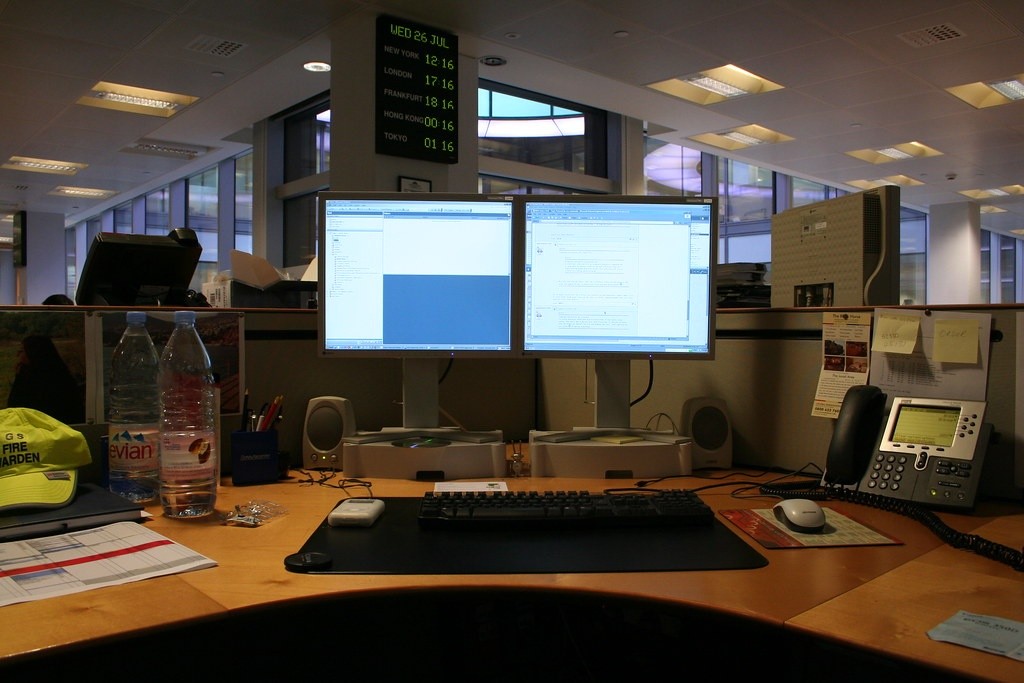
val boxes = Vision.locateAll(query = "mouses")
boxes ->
[773,498,827,533]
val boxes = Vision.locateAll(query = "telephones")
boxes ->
[821,385,995,510]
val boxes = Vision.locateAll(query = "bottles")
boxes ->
[158,310,219,519]
[108,311,161,504]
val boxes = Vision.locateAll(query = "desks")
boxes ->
[2,465,1023,682]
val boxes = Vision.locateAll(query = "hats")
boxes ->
[0,407,93,510]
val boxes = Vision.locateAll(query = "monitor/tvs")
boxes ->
[316,192,719,358]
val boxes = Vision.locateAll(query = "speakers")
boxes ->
[679,397,733,470]
[302,396,355,472]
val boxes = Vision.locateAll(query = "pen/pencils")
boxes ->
[242,388,283,430]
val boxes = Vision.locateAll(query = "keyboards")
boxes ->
[419,488,714,534]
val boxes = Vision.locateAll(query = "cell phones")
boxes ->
[329,496,385,529]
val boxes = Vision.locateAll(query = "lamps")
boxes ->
[957,183,1024,198]
[844,174,924,191]
[843,140,946,166]
[686,124,795,151]
[478,55,508,69]
[644,61,786,107]
[117,136,222,161]
[302,61,333,74]
[1008,227,1024,239]
[46,185,117,201]
[77,78,199,121]
[980,204,1008,216]
[944,75,1024,110]
[1,152,92,176]
